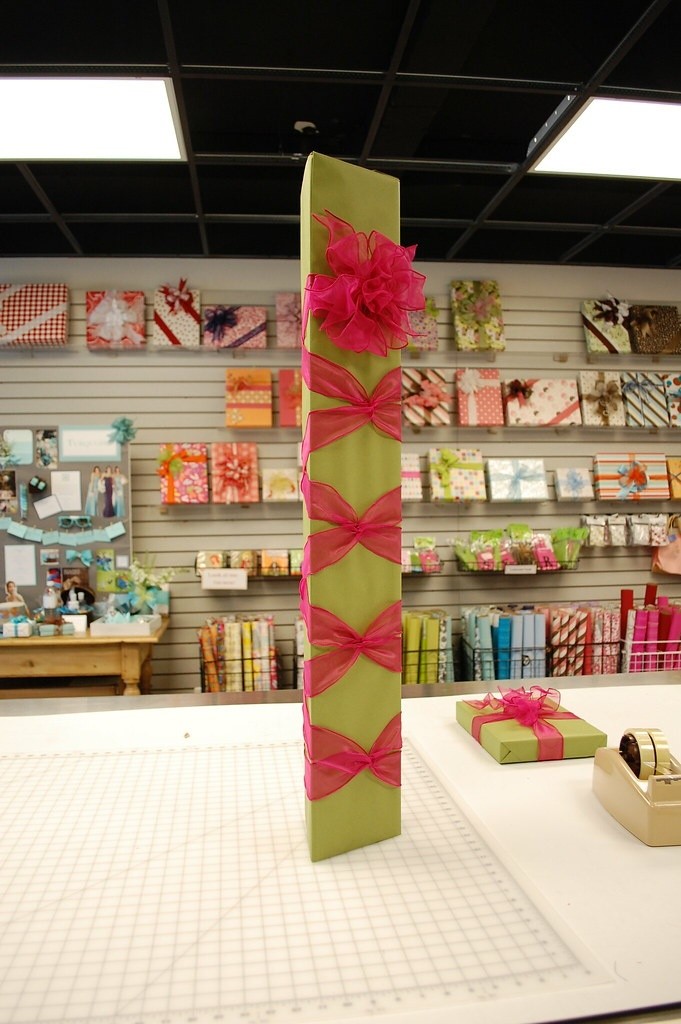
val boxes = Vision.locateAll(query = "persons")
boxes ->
[84,466,128,517]
[4,580,30,618]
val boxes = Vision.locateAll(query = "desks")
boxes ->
[0,670,681,1024]
[0,615,172,698]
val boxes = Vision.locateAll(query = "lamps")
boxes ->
[0,72,190,165]
[523,91,680,188]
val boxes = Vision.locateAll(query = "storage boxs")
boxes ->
[456,683,607,764]
[300,145,403,861]
[1,274,681,502]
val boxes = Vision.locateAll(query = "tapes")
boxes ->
[619,727,671,780]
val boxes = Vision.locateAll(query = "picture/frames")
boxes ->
[60,424,122,462]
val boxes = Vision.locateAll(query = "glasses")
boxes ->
[58,515,91,530]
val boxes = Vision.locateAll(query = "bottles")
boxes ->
[78,592,86,610]
[44,589,57,622]
[68,593,79,609]
